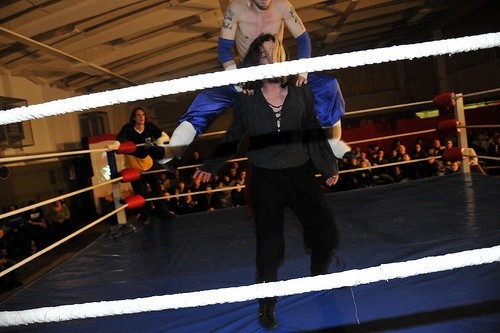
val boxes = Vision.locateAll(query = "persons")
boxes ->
[339,129,500,187]
[192,33,340,329]
[112,107,175,224]
[143,0,353,164]
[0,196,71,289]
[160,149,246,214]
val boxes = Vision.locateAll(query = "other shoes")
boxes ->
[327,139,356,159]
[146,141,180,174]
[257,302,278,330]
